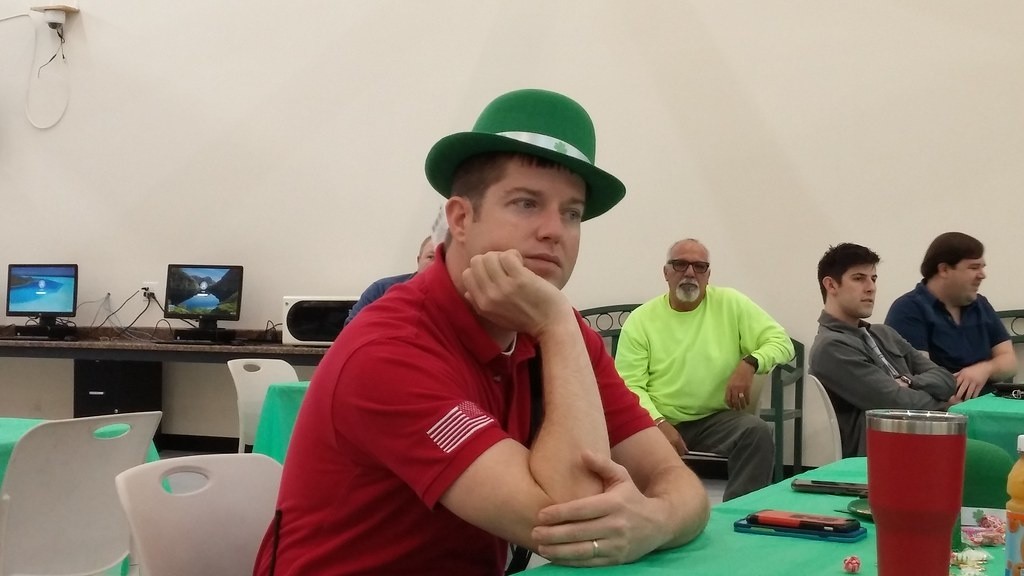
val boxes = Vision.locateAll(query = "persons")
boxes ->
[253,88,711,576]
[808,243,958,460]
[884,232,1019,400]
[614,238,796,502]
[343,235,438,327]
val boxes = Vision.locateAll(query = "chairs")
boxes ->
[226,357,300,454]
[807,373,842,461]
[1,411,163,575]
[114,455,283,575]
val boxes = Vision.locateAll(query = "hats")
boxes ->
[424,88,627,222]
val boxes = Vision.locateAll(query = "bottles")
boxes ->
[1005,435,1024,576]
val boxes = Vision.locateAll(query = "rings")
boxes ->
[592,540,598,557]
[739,393,744,398]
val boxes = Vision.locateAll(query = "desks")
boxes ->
[248,380,311,465]
[946,394,1024,461]
[1,418,169,576]
[504,456,1006,576]
[1,337,327,366]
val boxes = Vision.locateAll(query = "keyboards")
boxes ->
[153,340,218,344]
[0,336,57,340]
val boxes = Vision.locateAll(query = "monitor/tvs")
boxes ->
[6,264,78,326]
[164,263,243,330]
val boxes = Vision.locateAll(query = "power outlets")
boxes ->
[142,282,159,300]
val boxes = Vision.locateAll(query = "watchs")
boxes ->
[743,355,759,372]
[901,375,912,387]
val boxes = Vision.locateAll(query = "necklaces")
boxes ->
[503,336,516,356]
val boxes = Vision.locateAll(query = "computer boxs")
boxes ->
[16,326,76,339]
[174,329,237,343]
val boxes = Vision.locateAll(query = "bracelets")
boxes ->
[657,419,665,426]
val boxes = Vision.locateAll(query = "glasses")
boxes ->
[667,259,709,273]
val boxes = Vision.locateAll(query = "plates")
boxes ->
[847,498,872,520]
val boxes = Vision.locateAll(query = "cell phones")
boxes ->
[791,478,868,497]
[734,508,868,543]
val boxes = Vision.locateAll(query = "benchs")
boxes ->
[576,303,805,487]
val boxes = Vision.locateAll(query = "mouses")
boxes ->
[232,340,245,346]
[65,336,78,341]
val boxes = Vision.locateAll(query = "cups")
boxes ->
[865,408,966,576]
